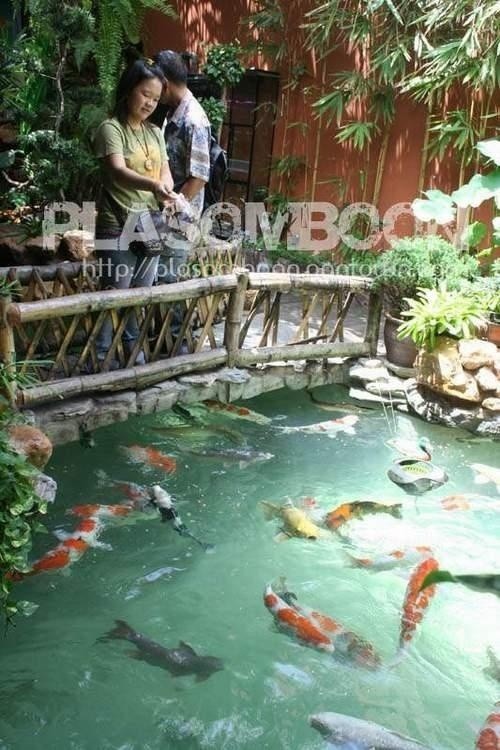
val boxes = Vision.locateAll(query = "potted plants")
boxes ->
[367,235,481,368]
[485,257,500,347]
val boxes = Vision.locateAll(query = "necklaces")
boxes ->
[124,117,155,172]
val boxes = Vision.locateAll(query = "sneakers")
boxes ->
[125,348,146,366]
[148,333,194,355]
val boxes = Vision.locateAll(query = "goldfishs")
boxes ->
[8,395,499,749]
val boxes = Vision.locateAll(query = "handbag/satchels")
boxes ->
[119,210,170,257]
[205,139,227,210]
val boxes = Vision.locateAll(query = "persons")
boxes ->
[86,56,179,375]
[143,48,212,355]
